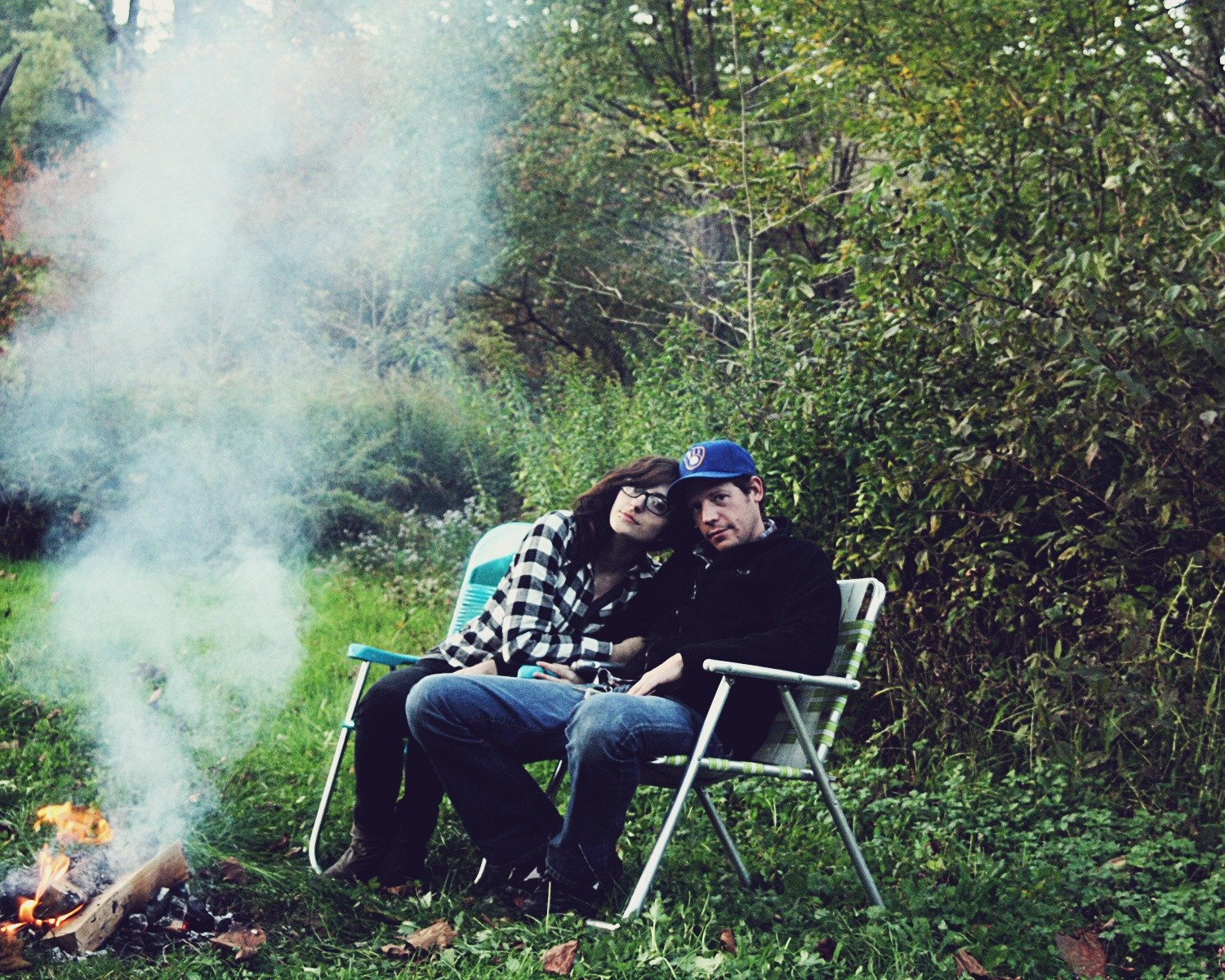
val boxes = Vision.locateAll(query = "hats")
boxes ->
[666,440,758,517]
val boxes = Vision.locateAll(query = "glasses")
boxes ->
[621,476,672,516]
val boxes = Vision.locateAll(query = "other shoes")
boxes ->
[364,846,439,894]
[468,863,535,912]
[322,837,374,878]
[522,850,619,928]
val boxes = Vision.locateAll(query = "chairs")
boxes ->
[581,575,888,930]
[307,520,571,889]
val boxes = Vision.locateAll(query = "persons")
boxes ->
[405,440,843,917]
[321,457,682,895]
